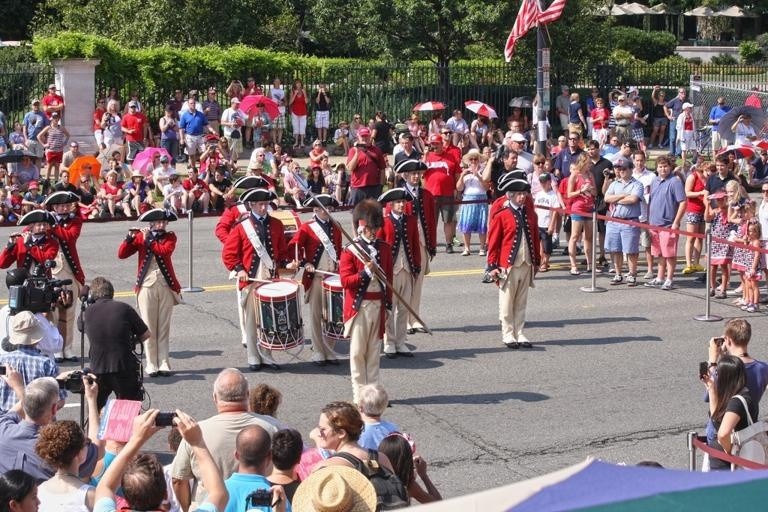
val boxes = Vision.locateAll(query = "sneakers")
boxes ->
[704,274,767,313]
[661,279,674,290]
[643,277,665,288]
[627,276,637,288]
[452,236,465,247]
[609,275,624,285]
[568,265,580,276]
[445,244,454,254]
[643,271,655,280]
[461,249,471,257]
[682,264,707,274]
[551,237,629,273]
[478,247,487,257]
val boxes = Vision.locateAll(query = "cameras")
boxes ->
[210,144,218,152]
[602,167,616,179]
[714,337,724,347]
[155,413,177,426]
[0,366,6,374]
[354,143,366,147]
[252,489,281,506]
[57,367,95,393]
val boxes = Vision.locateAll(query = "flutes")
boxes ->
[58,212,70,216]
[128,229,163,232]
[10,231,46,238]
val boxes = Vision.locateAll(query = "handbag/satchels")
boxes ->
[727,418,768,472]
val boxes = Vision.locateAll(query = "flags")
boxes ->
[503,0,567,64]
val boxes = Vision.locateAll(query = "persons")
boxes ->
[700,316,768,407]
[482,179,541,350]
[699,353,757,474]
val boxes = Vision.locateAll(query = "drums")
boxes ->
[268,208,304,274]
[320,274,352,341]
[253,278,305,351]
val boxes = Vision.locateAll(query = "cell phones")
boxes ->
[699,362,708,379]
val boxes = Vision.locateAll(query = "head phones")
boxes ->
[87,288,95,304]
[7,270,23,285]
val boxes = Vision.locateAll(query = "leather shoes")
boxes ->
[158,371,171,377]
[263,363,280,370]
[416,328,428,333]
[327,359,340,365]
[249,365,261,371]
[504,342,519,349]
[314,361,328,366]
[405,328,415,334]
[519,342,532,348]
[397,352,414,357]
[150,372,158,378]
[55,358,63,363]
[64,357,78,362]
[385,352,397,359]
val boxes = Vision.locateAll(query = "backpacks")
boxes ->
[325,448,411,510]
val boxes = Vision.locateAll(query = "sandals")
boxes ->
[539,262,549,273]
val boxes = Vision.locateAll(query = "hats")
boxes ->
[354,201,385,238]
[376,187,414,205]
[7,309,47,345]
[0,78,694,192]
[17,209,56,226]
[137,208,178,223]
[291,464,378,511]
[302,194,341,208]
[44,190,81,205]
[237,187,279,204]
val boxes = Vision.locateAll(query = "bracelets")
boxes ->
[706,360,717,369]
